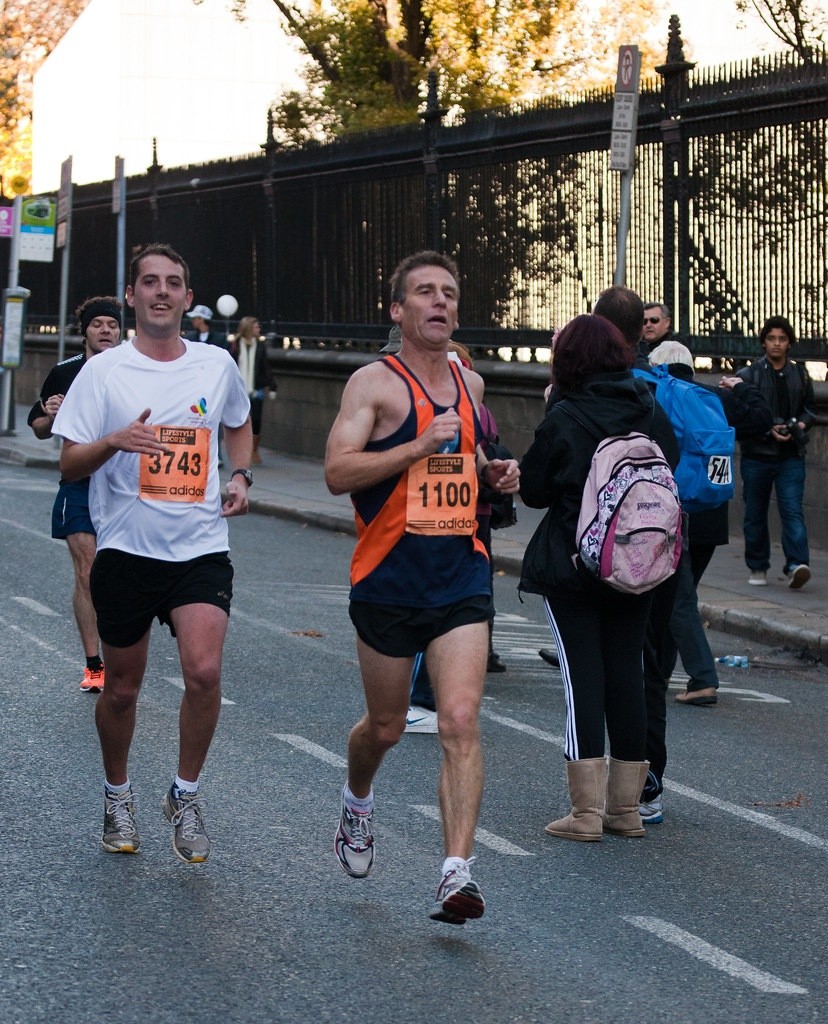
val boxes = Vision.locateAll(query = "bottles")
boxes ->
[719,654,748,668]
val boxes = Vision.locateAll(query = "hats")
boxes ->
[185,305,213,320]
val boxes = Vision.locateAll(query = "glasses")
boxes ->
[642,316,665,325]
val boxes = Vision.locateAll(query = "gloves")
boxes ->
[269,391,277,400]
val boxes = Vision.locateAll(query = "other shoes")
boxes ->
[403,704,439,734]
[748,568,767,585]
[218,457,224,466]
[674,687,717,704]
[639,794,664,824]
[787,564,811,589]
[539,649,559,668]
[486,654,507,673]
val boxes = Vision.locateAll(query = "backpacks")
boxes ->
[630,364,735,512]
[553,392,689,594]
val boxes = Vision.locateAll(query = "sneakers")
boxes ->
[429,856,485,925]
[333,781,375,878]
[80,663,105,693]
[161,781,211,863]
[101,785,141,854]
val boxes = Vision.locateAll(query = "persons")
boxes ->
[325,251,523,924]
[183,305,234,468]
[518,287,729,842]
[50,245,254,863]
[27,298,123,693]
[224,316,278,464]
[726,316,815,589]
[378,327,514,735]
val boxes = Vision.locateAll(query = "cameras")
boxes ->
[780,416,810,448]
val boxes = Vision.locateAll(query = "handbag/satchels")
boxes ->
[484,442,517,530]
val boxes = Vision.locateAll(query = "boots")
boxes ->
[253,440,261,464]
[544,758,607,842]
[602,755,650,836]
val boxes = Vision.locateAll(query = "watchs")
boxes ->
[232,469,253,486]
